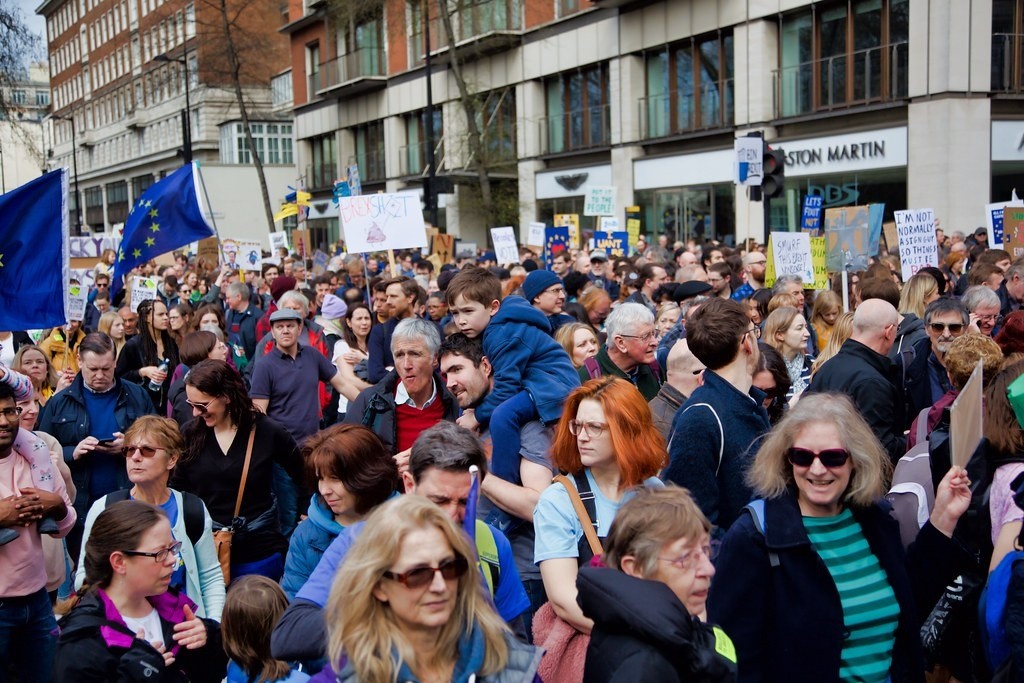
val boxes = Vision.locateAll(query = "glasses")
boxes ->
[185,393,222,413]
[659,538,723,566]
[543,287,565,295]
[787,446,852,467]
[759,387,779,398]
[568,419,610,438]
[120,445,169,457]
[0,406,23,421]
[97,283,108,287]
[928,321,965,334]
[982,314,1004,323]
[620,329,661,341]
[741,324,763,345]
[386,551,469,589]
[122,541,183,562]
[749,261,767,265]
[180,290,192,294]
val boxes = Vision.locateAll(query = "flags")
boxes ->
[0,168,72,333]
[110,161,217,308]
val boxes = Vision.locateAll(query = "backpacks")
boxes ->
[883,406,934,548]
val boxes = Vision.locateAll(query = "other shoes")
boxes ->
[37,516,60,534]
[0,528,19,547]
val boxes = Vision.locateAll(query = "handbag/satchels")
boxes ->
[213,526,232,587]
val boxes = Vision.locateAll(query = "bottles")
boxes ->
[149,358,169,391]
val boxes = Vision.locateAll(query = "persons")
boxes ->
[0,228,1024,683]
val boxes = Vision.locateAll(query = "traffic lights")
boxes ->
[762,150,787,198]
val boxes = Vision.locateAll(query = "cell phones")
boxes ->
[97,438,114,446]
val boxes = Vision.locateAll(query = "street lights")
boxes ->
[154,54,193,164]
[50,114,80,236]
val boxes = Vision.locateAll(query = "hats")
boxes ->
[270,308,302,322]
[271,225,987,301]
[321,293,348,320]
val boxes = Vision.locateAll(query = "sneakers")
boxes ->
[485,505,525,539]
